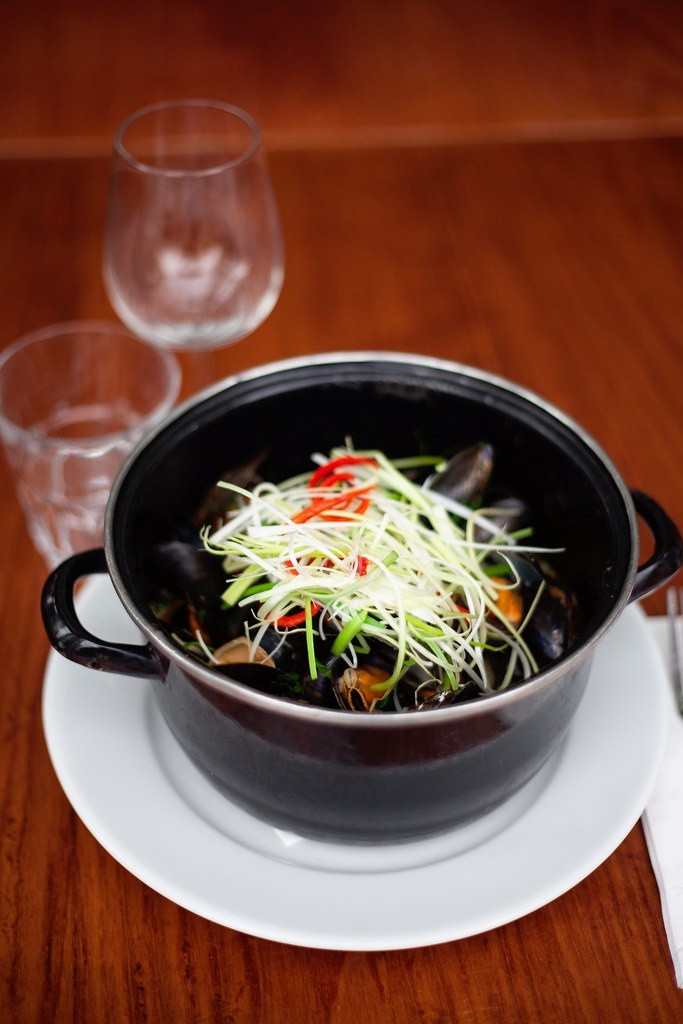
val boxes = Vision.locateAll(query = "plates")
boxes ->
[42,575,672,950]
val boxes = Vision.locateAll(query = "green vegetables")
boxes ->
[199,437,567,705]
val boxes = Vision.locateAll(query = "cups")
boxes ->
[102,101,286,348]
[0,321,180,568]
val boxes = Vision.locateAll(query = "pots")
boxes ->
[41,352,683,845]
